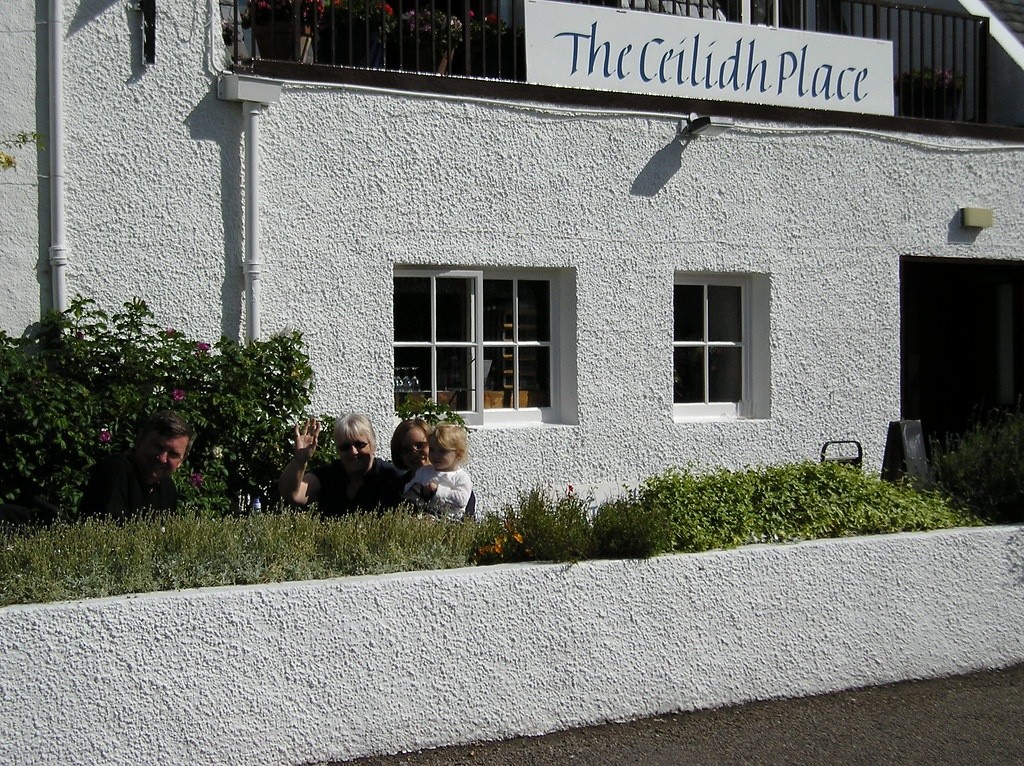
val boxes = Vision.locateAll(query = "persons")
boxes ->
[83,411,194,527]
[281,412,476,519]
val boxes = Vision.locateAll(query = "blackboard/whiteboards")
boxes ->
[899,419,928,482]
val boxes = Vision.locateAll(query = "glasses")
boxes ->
[401,442,428,456]
[340,441,367,450]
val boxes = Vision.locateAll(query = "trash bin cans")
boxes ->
[820,441,864,468]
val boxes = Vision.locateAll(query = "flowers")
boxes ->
[242,0,508,49]
[895,67,965,93]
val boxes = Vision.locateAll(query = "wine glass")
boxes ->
[402,367,411,392]
[394,367,403,392]
[411,367,420,391]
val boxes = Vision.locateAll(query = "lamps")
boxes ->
[687,116,733,138]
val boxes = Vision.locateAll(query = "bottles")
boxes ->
[251,498,262,517]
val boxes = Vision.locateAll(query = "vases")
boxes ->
[903,89,959,119]
[398,40,456,74]
[289,25,332,64]
[345,31,383,67]
